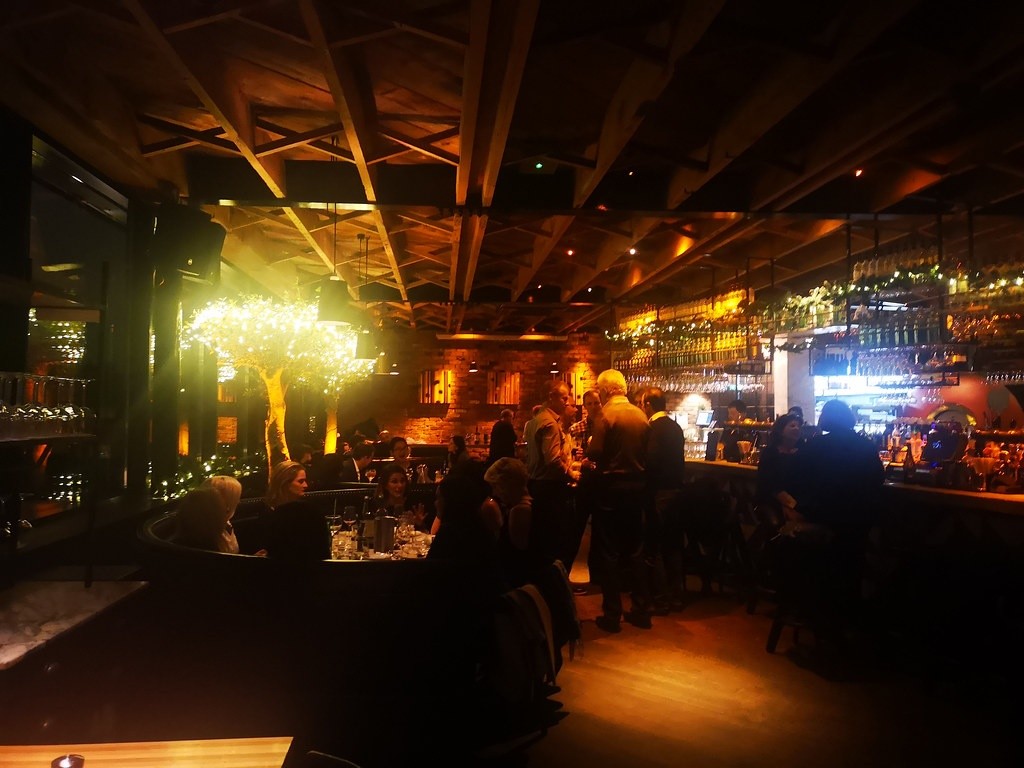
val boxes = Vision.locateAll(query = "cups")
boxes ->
[751,451,760,465]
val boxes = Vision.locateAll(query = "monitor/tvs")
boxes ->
[696,410,714,426]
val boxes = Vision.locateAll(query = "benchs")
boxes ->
[135,487,451,699]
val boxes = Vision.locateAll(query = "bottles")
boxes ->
[825,339,974,374]
[362,539,374,555]
[873,394,908,418]
[984,370,1024,384]
[333,542,357,560]
[614,324,656,369]
[464,427,491,445]
[858,321,940,346]
[860,423,957,482]
[657,316,762,366]
[854,244,939,284]
[945,255,1024,343]
[620,303,657,331]
[658,288,763,323]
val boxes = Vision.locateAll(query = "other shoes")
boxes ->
[624,611,651,627]
[596,616,621,632]
[573,588,588,595]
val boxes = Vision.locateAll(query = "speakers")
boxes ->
[148,208,227,286]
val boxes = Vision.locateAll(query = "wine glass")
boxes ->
[872,379,945,406]
[857,354,920,378]
[624,364,764,392]
[326,515,343,556]
[738,441,750,464]
[344,507,358,544]
[684,442,707,458]
[879,451,894,486]
[0,370,97,436]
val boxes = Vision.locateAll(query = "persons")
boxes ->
[634,386,685,614]
[489,409,518,458]
[756,400,886,549]
[264,501,332,560]
[586,370,655,633]
[727,400,747,420]
[172,476,267,556]
[266,461,308,510]
[523,380,602,595]
[368,465,428,529]
[289,428,468,482]
[417,454,530,588]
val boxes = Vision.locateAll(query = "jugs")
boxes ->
[375,517,400,554]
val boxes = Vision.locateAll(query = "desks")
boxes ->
[0,554,150,670]
[406,441,529,451]
[0,736,293,768]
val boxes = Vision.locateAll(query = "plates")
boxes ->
[362,555,392,559]
[398,550,427,558]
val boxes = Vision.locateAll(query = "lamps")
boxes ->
[549,362,559,374]
[315,136,399,375]
[469,360,478,373]
